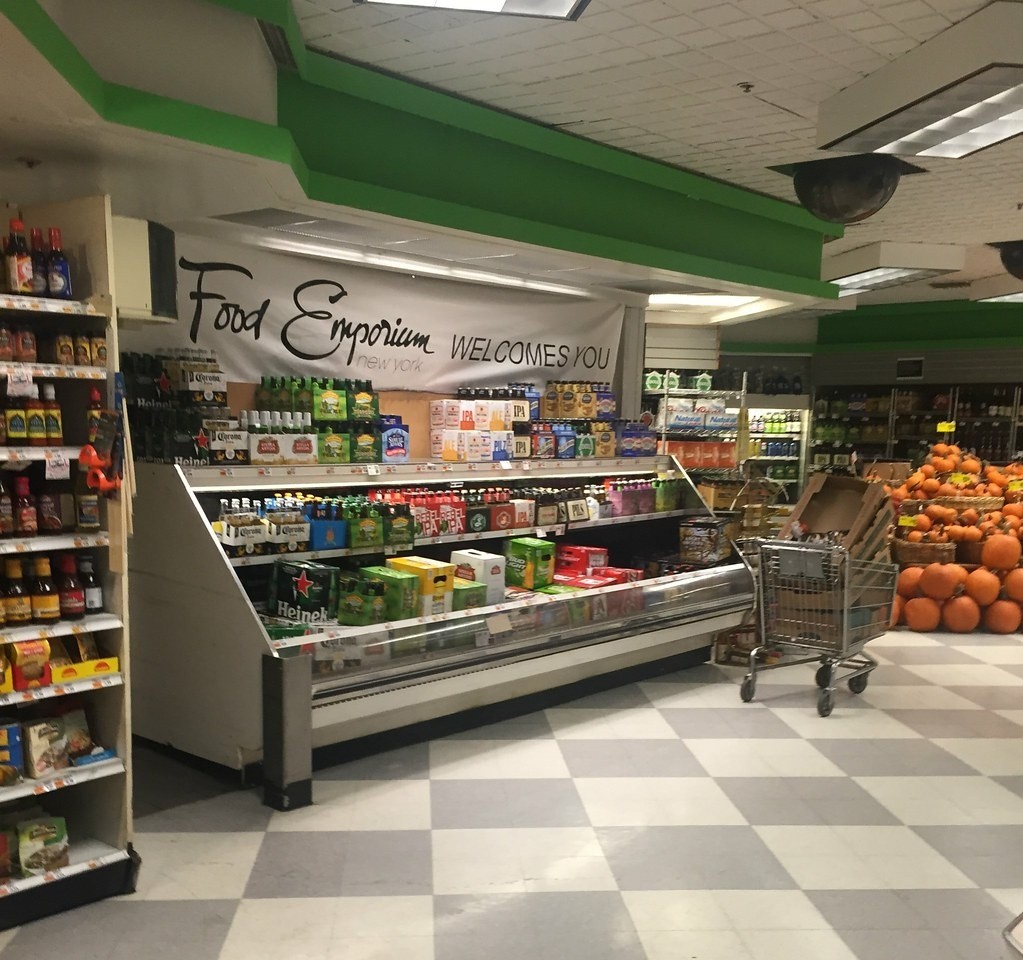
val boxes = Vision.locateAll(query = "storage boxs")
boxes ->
[222,470,897,675]
[178,370,644,673]
[22,716,70,779]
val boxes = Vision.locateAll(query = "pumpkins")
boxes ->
[860,441,1023,635]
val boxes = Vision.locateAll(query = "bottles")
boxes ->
[458,380,611,400]
[0,382,104,447]
[807,386,1023,477]
[121,347,219,372]
[218,476,746,537]
[644,364,803,395]
[749,439,797,456]
[646,557,717,578]
[530,419,645,434]
[716,625,779,666]
[128,405,231,464]
[751,411,801,433]
[339,576,384,596]
[0,218,74,300]
[0,548,103,629]
[0,461,101,539]
[240,409,378,434]
[261,375,374,394]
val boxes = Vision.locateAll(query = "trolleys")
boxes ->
[729,535,900,718]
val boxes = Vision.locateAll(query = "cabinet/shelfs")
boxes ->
[0,193,141,932]
[129,454,757,811]
[643,370,1023,523]
